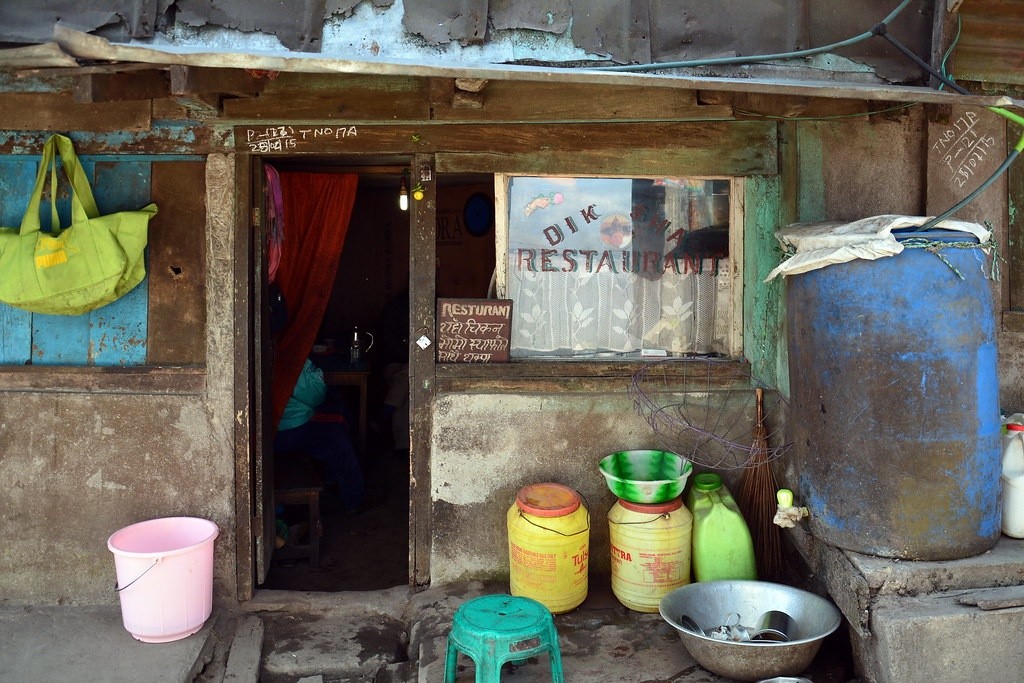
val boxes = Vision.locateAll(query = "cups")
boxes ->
[753,611,798,642]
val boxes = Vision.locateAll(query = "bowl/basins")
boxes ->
[599,448,694,503]
[659,579,842,682]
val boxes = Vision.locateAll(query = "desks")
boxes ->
[321,360,372,451]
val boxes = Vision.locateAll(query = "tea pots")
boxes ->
[344,326,374,354]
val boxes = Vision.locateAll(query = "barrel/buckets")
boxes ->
[507,482,591,614]
[105,516,222,643]
[776,216,1006,560]
[606,497,695,614]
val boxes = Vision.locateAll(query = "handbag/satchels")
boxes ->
[0,134,158,315]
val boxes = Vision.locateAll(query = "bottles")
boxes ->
[686,471,759,584]
[997,421,1024,539]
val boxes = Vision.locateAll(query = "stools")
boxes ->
[444,594,565,683]
[275,458,321,570]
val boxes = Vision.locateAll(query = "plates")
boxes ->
[677,614,705,635]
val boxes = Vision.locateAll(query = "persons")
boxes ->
[271,355,409,515]
